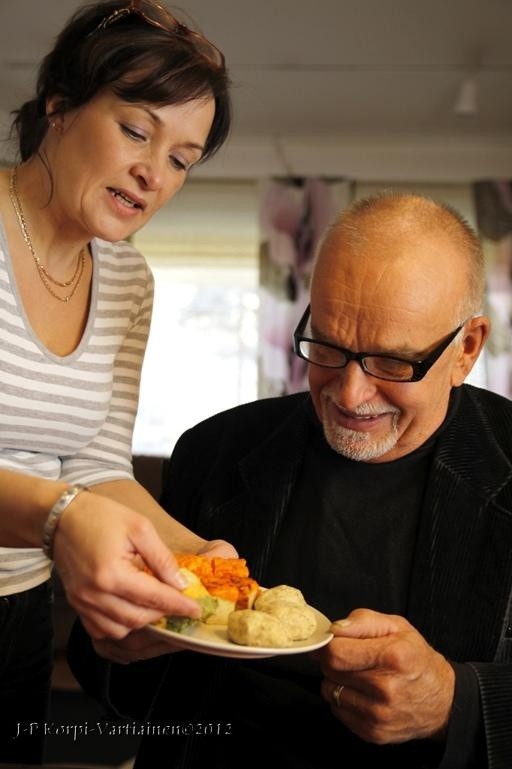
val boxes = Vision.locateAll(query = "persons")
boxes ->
[0,1,239,768]
[61,189,512,768]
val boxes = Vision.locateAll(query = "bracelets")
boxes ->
[40,484,88,561]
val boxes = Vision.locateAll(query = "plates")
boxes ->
[144,584,336,661]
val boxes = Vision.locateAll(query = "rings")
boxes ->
[328,683,346,711]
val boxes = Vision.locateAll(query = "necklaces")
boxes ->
[8,161,86,304]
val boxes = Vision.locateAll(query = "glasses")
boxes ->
[294,304,469,382]
[82,0,225,71]
